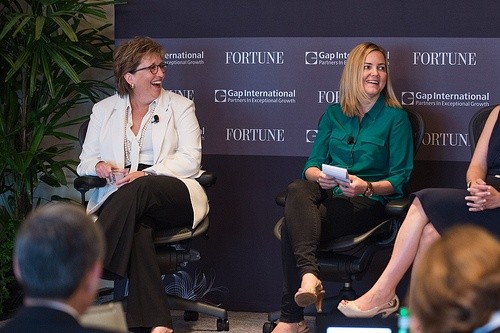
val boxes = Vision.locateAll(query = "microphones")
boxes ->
[154,115,159,122]
[348,137,354,144]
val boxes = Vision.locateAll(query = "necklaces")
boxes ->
[124,106,155,163]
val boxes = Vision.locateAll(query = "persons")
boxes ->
[0,201,124,333]
[76,34,210,333]
[264,41,414,333]
[336,103,500,333]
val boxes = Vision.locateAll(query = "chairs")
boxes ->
[262,109,425,333]
[74,120,230,331]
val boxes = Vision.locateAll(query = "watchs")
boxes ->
[364,182,373,198]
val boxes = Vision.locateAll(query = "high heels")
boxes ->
[296,319,310,332]
[339,294,399,320]
[295,279,325,313]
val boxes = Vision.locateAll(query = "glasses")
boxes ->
[130,62,168,74]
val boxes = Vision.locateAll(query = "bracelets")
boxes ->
[466,179,472,188]
